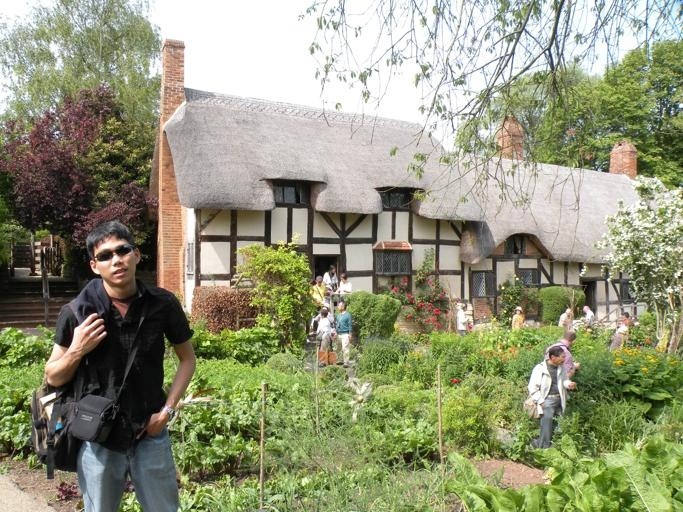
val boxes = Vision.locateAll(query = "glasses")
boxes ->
[95,245,135,261]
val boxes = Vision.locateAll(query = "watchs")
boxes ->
[159,405,175,422]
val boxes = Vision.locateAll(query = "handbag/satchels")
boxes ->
[31,384,81,472]
[524,397,544,419]
[70,395,115,444]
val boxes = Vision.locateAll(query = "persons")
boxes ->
[512,308,536,330]
[457,303,469,334]
[559,308,571,326]
[310,265,353,368]
[612,313,634,347]
[44,217,196,512]
[584,306,594,328]
[528,331,580,449]
